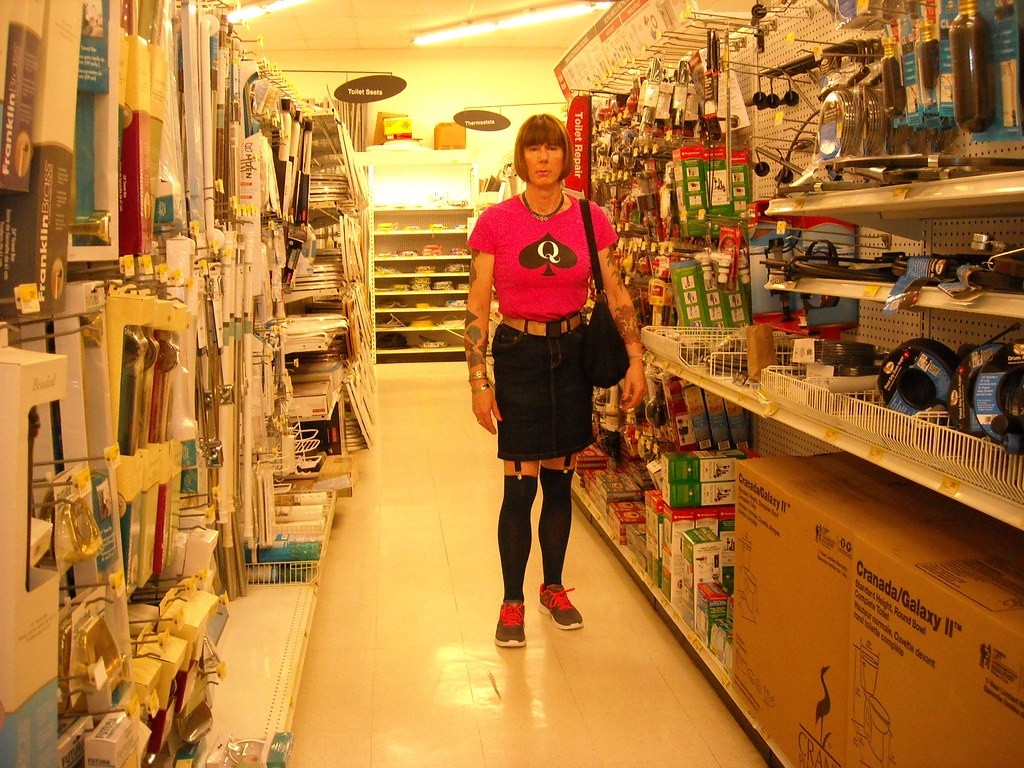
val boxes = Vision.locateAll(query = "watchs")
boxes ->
[632,354,647,365]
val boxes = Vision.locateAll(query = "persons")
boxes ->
[465,113,648,647]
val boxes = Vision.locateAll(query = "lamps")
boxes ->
[412,0,615,46]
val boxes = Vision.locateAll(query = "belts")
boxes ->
[502,312,581,337]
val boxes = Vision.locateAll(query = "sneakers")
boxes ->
[495,600,526,647]
[539,582,583,629]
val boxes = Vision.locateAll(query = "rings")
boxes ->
[477,420,481,423]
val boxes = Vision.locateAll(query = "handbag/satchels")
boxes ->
[579,198,630,387]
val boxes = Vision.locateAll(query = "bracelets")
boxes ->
[468,371,489,383]
[471,383,490,393]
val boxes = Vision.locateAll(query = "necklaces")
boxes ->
[522,188,564,221]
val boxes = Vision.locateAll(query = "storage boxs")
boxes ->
[643,139,1024,768]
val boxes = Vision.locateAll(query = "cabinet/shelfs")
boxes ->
[642,176,1024,768]
[363,159,481,365]
